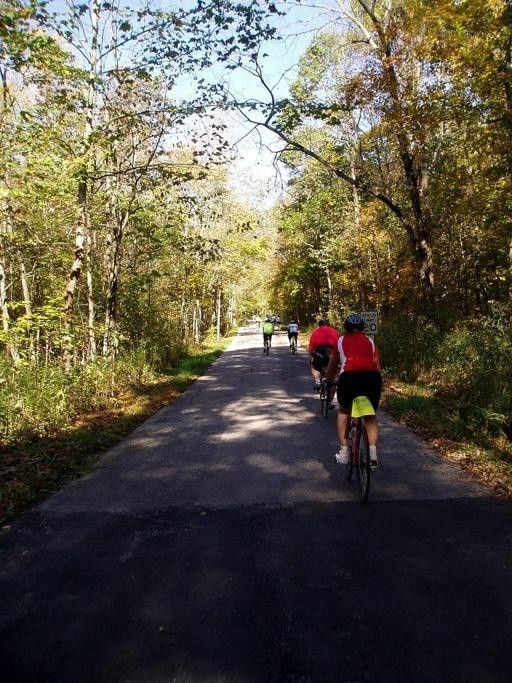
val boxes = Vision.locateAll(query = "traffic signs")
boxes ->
[360,310,378,333]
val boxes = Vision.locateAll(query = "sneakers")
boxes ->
[328,402,335,409]
[370,460,384,472]
[335,450,349,464]
[313,383,321,389]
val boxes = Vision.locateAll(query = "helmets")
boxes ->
[344,315,363,329]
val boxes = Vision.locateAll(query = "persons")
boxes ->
[287,319,299,352]
[324,311,385,471]
[306,318,342,410]
[263,318,274,352]
[255,314,282,327]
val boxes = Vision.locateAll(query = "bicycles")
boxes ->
[344,394,376,504]
[263,336,270,355]
[291,335,297,355]
[309,359,335,418]
[256,321,281,331]
